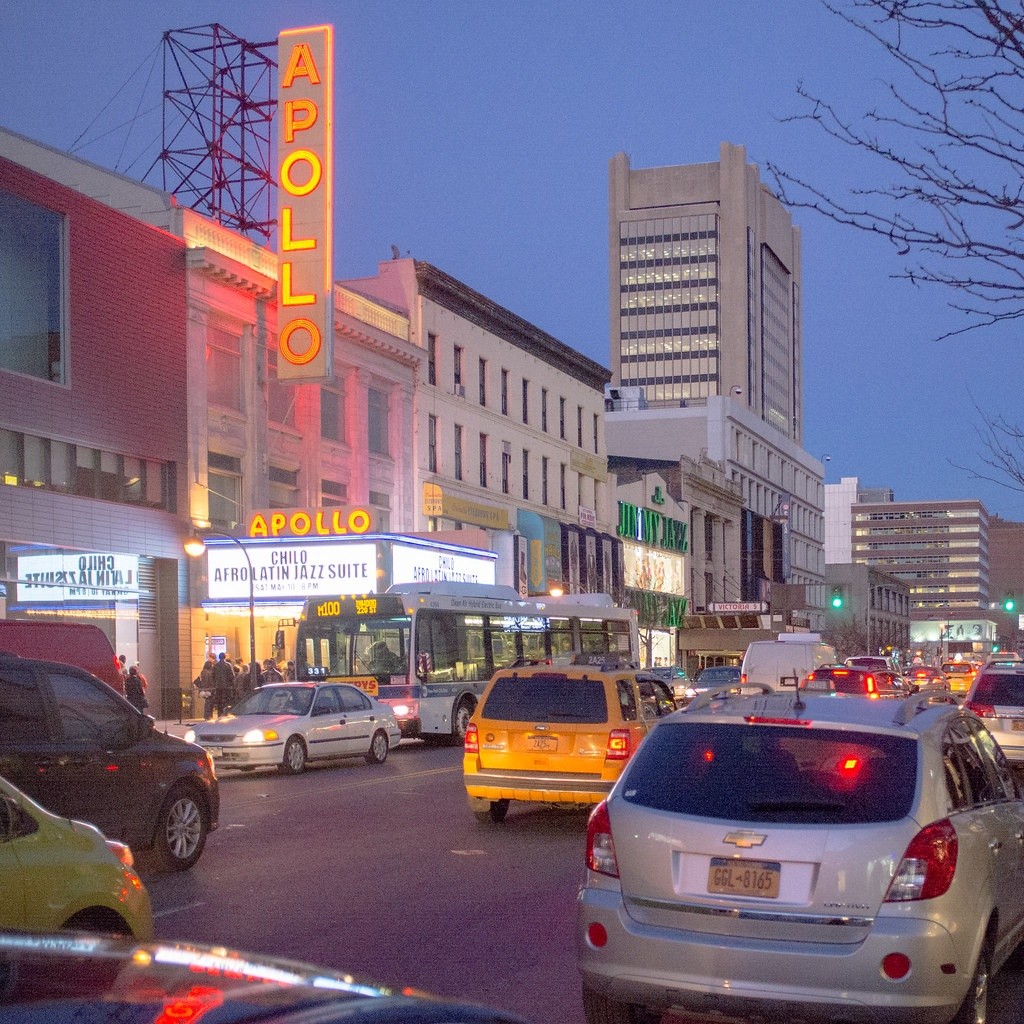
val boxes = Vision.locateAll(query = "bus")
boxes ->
[276,576,641,747]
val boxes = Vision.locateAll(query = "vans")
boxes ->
[0,618,127,700]
[740,633,841,695]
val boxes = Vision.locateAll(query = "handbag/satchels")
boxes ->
[193,677,201,688]
[135,698,149,709]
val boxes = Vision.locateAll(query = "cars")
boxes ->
[639,666,692,704]
[798,655,1024,768]
[0,770,155,944]
[684,665,742,706]
[182,681,402,777]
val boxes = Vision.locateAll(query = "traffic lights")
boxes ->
[833,585,841,607]
[1006,590,1015,611]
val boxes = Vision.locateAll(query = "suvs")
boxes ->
[576,684,1024,1024]
[0,651,221,875]
[463,657,680,824]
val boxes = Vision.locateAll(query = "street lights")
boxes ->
[867,584,898,656]
[183,529,257,691]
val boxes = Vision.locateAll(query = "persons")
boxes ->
[604,543,610,594]
[587,541,596,594]
[551,637,617,657]
[654,657,668,666]
[641,558,666,588]
[940,628,953,640]
[619,549,624,601]
[370,638,431,672]
[956,628,968,641]
[695,665,703,678]
[330,646,359,674]
[119,655,128,675]
[136,666,148,693]
[570,534,578,594]
[199,652,295,719]
[125,665,144,714]
[969,624,982,640]
